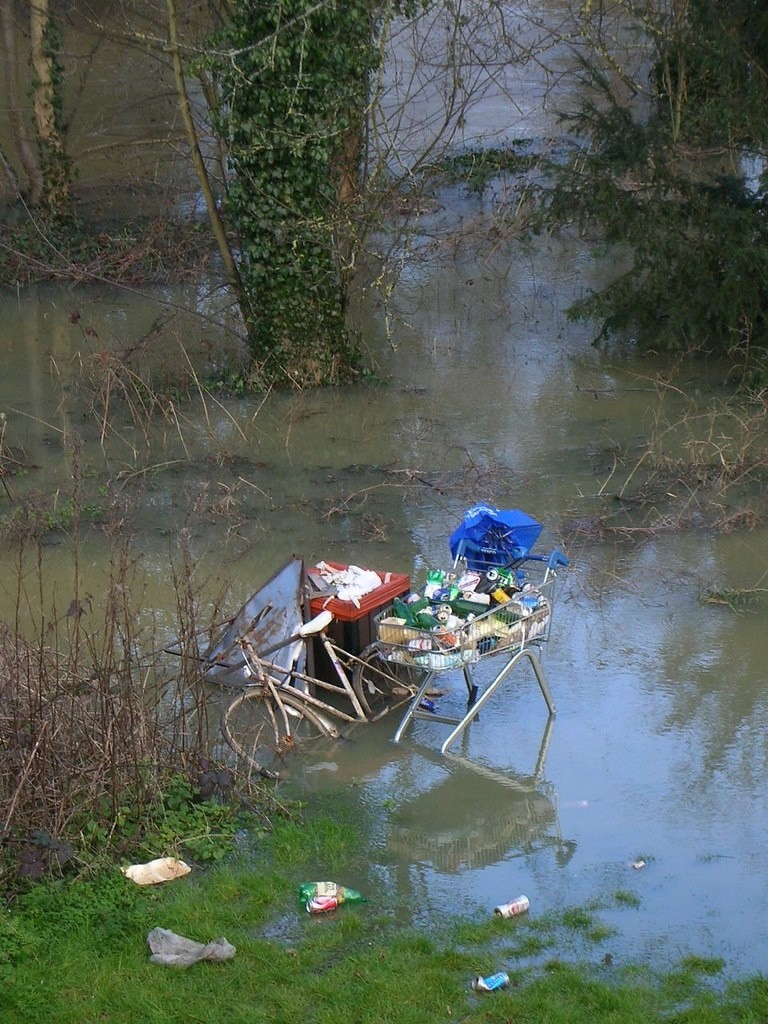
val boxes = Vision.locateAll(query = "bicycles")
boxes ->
[213,599,450,780]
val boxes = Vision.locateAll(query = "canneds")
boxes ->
[433,568,530,648]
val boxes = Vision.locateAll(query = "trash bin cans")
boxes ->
[300,561,410,693]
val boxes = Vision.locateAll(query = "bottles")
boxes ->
[299,881,371,904]
[380,568,545,665]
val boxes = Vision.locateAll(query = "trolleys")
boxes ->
[371,547,571,793]
